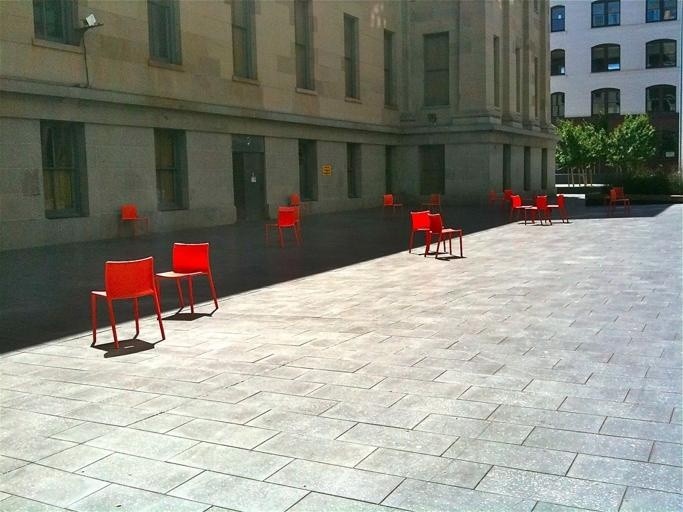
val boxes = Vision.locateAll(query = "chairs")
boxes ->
[488,188,568,226]
[90,255,164,350]
[383,194,403,216]
[289,192,298,207]
[408,210,430,252]
[118,203,149,239]
[265,207,301,249]
[608,186,630,217]
[421,193,439,213]
[423,214,462,259]
[155,241,218,320]
